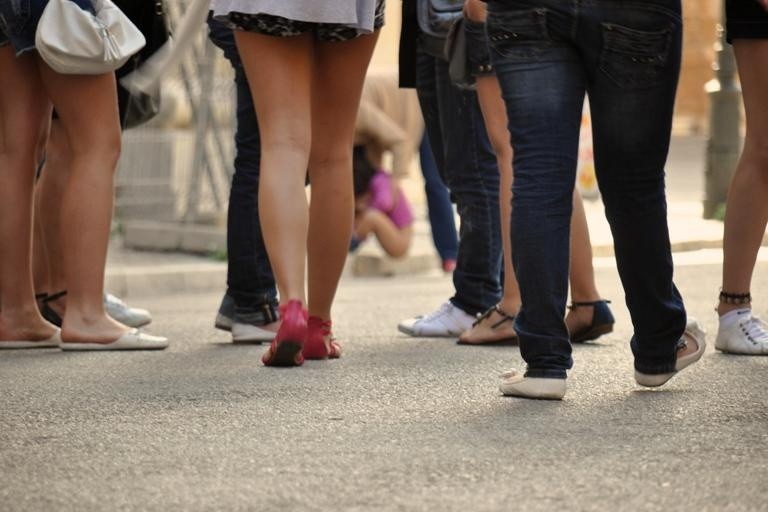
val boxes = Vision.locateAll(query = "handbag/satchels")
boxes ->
[34,0,145,76]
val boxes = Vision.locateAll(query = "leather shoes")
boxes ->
[216,313,234,329]
[634,321,706,387]
[499,368,567,401]
[232,323,276,344]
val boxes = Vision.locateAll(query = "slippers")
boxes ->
[59,327,169,351]
[0,326,62,348]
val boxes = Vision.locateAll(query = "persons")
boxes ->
[1,1,768,401]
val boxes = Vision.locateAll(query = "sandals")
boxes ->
[456,304,519,345]
[303,316,342,359]
[42,290,67,326]
[262,300,309,366]
[566,300,614,342]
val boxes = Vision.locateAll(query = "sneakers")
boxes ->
[715,316,767,355]
[106,294,151,328]
[398,302,477,336]
